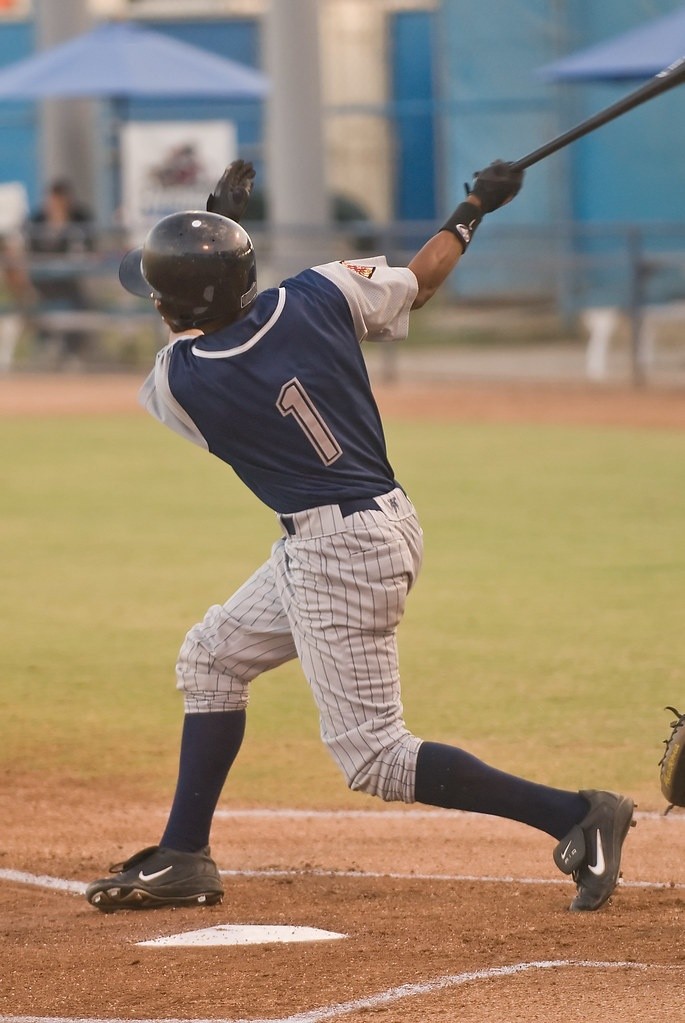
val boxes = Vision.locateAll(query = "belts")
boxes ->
[280,478,407,536]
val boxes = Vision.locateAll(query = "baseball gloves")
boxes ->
[655,703,684,816]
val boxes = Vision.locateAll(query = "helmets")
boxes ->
[117,211,258,332]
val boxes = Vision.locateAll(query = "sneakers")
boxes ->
[86,845,226,913]
[553,789,638,910]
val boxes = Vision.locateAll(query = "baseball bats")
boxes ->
[508,57,685,172]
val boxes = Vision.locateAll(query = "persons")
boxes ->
[85,160,641,912]
[23,176,95,364]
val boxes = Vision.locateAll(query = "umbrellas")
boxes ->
[0,19,272,224]
[539,13,685,80]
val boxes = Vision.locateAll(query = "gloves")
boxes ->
[468,160,524,213]
[207,160,256,222]
